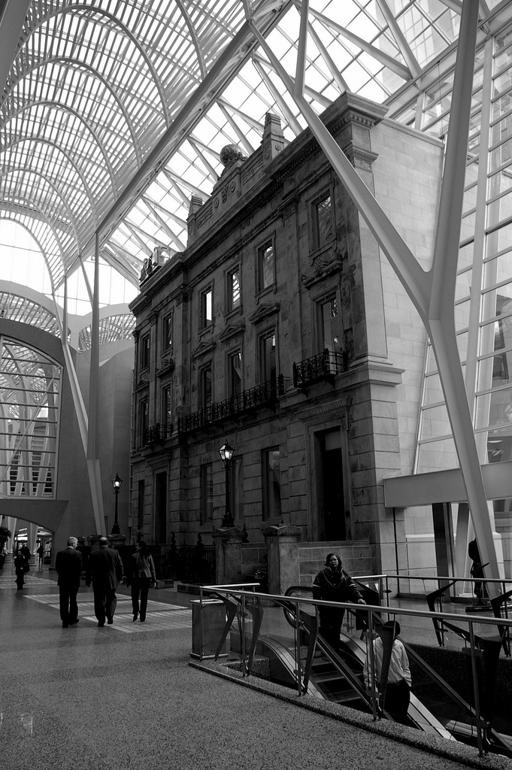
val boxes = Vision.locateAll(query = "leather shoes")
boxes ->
[98,616,113,626]
[133,614,145,622]
[62,619,79,627]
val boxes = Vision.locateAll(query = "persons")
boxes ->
[311,552,368,662]
[363,618,413,725]
[1,532,158,629]
[468,536,489,606]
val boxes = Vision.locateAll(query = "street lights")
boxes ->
[218,438,237,529]
[111,472,121,534]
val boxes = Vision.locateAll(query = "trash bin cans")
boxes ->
[189,598,229,659]
